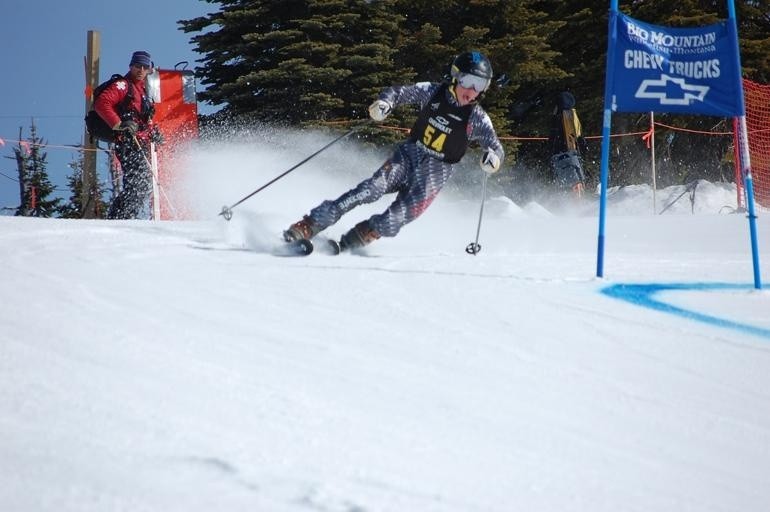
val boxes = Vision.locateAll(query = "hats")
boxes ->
[129,51,151,67]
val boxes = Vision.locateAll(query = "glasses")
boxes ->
[451,67,489,93]
[135,63,149,69]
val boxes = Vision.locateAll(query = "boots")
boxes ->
[288,216,320,243]
[345,219,381,252]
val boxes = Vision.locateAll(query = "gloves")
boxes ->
[368,100,392,122]
[479,150,501,175]
[112,120,138,136]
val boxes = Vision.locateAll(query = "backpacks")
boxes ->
[84,74,133,143]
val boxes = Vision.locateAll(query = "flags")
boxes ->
[142,68,199,223]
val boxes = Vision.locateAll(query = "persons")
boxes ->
[90,49,167,221]
[281,51,506,257]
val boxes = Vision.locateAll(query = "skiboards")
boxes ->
[282,238,342,256]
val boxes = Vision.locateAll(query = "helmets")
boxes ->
[448,52,493,101]
